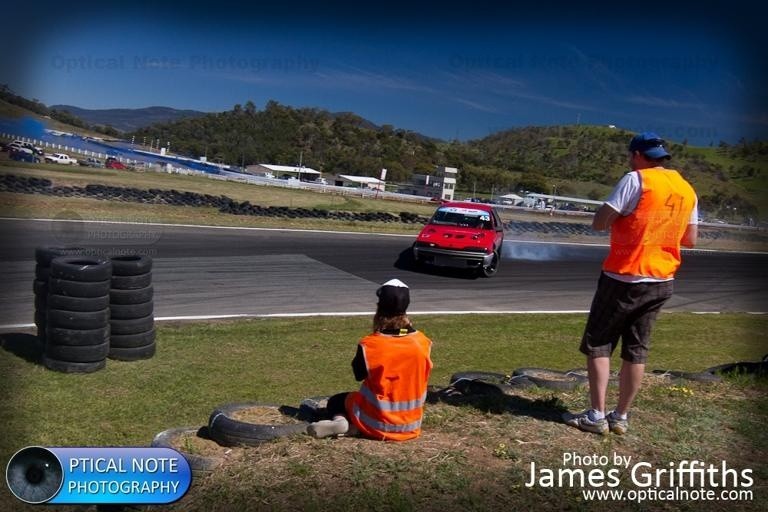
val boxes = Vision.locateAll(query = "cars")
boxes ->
[534,201,578,211]
[502,199,512,205]
[413,199,503,276]
[7,141,43,163]
[107,157,127,170]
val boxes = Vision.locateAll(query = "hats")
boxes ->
[377,280,411,316]
[629,132,675,161]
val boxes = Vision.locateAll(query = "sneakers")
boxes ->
[563,410,628,435]
[307,417,360,438]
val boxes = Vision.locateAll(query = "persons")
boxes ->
[307,278,432,442]
[561,132,699,436]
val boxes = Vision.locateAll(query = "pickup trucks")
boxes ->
[80,157,104,168]
[45,153,78,165]
